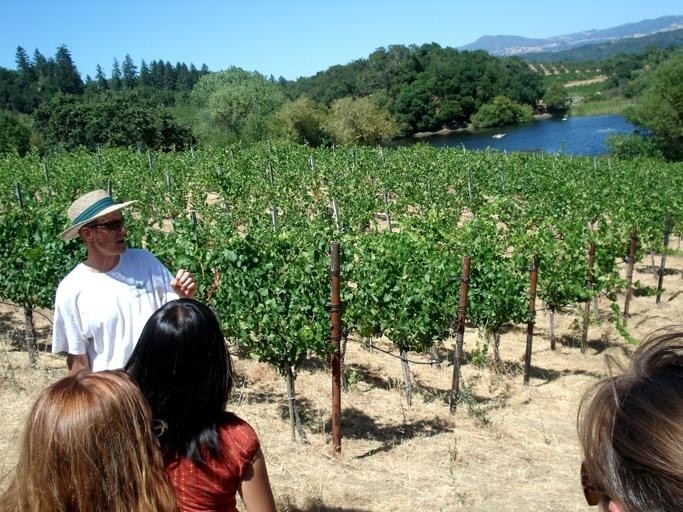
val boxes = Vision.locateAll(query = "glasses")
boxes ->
[580,459,609,506]
[100,215,126,233]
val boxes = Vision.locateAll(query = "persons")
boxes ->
[52,189,199,374]
[575,324,683,512]
[1,298,277,512]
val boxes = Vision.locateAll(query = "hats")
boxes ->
[59,189,138,241]
